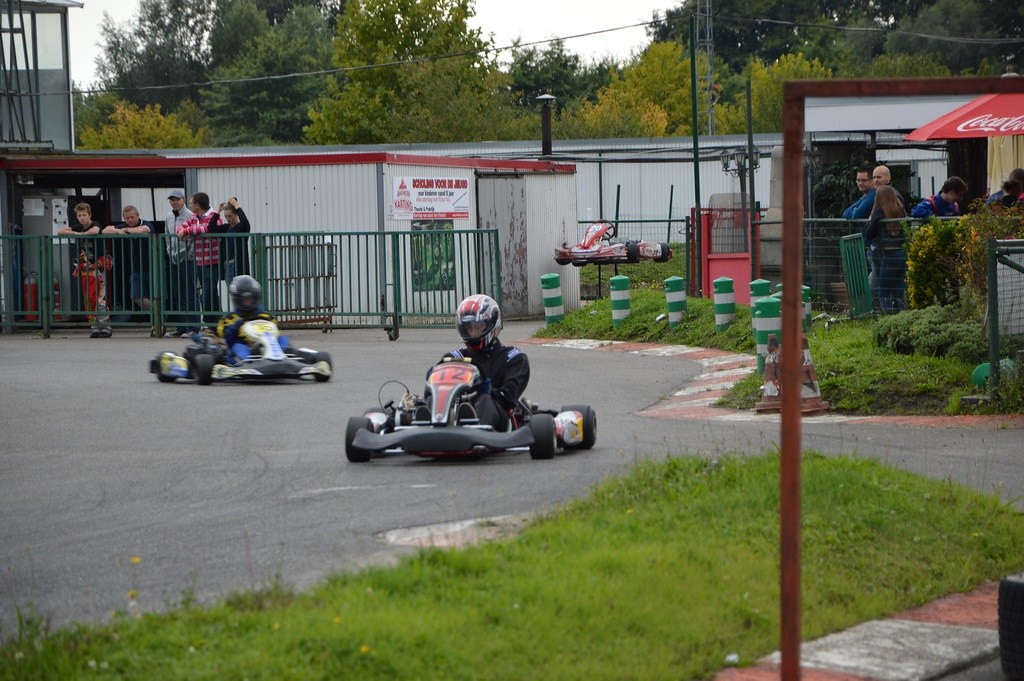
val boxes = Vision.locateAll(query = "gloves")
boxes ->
[475,377,491,394]
[224,320,244,337]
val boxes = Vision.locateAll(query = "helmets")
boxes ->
[230,275,262,314]
[454,294,503,350]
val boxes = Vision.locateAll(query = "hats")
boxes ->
[167,191,184,199]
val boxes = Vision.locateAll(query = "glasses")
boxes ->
[856,178,871,183]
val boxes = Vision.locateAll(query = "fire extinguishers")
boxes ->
[23,272,39,321]
[54,272,61,320]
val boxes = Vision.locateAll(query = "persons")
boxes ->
[912,176,968,303]
[862,185,908,315]
[59,203,113,337]
[842,167,877,219]
[176,193,223,330]
[208,196,250,275]
[863,166,904,308]
[985,167,1024,208]
[217,275,288,359]
[165,190,195,338]
[424,294,530,432]
[102,205,166,311]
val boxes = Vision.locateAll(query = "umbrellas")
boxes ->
[902,93,1024,142]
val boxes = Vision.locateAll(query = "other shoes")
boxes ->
[181,331,196,337]
[164,330,184,338]
[90,330,112,338]
